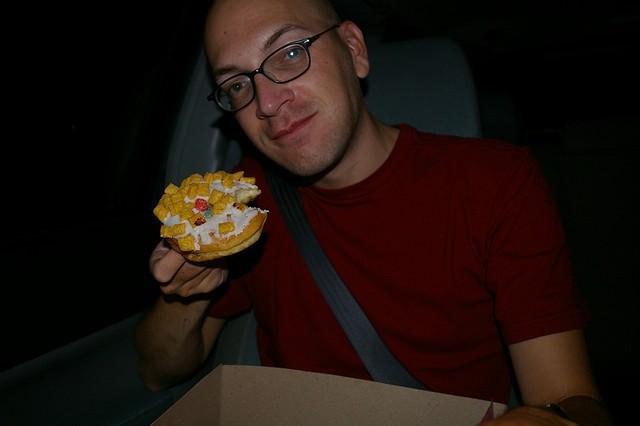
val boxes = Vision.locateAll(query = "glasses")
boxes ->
[206,25,339,113]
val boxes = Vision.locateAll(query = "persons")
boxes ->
[128,1,609,425]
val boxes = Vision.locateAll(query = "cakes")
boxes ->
[152,170,269,262]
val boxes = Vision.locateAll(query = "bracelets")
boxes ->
[539,404,571,419]
